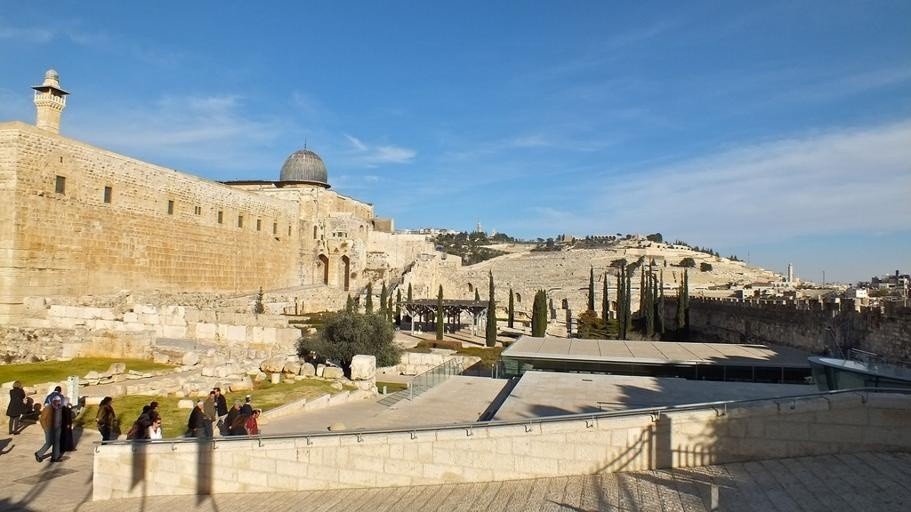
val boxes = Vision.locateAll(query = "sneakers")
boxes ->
[35,452,62,462]
[9,431,19,435]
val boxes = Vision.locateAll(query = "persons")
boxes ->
[95,396,117,445]
[186,387,261,436]
[125,399,163,442]
[5,381,77,463]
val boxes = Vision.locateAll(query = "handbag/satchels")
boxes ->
[217,420,230,435]
[110,426,121,440]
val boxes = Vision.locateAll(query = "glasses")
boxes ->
[157,422,160,424]
